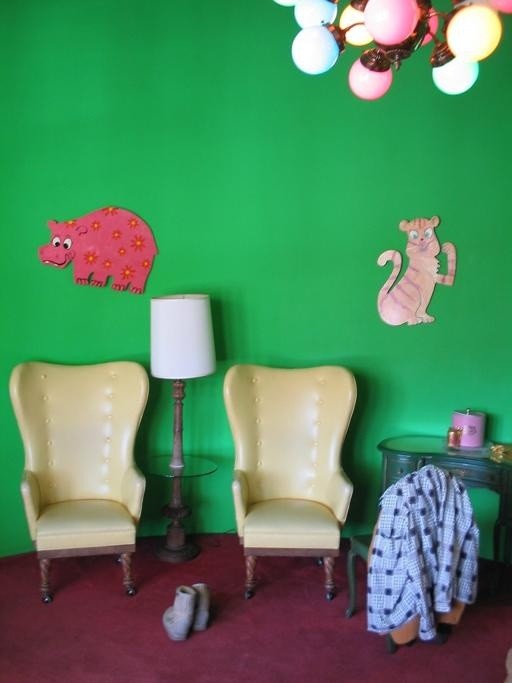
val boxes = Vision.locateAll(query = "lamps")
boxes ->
[150,294,216,467]
[277,1,512,99]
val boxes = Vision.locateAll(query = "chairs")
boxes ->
[223,364,357,600]
[346,465,474,653]
[10,361,148,602]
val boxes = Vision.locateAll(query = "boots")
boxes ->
[163,586,195,642]
[195,582,209,630]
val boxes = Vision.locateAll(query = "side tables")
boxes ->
[139,456,217,563]
[377,434,512,608]
